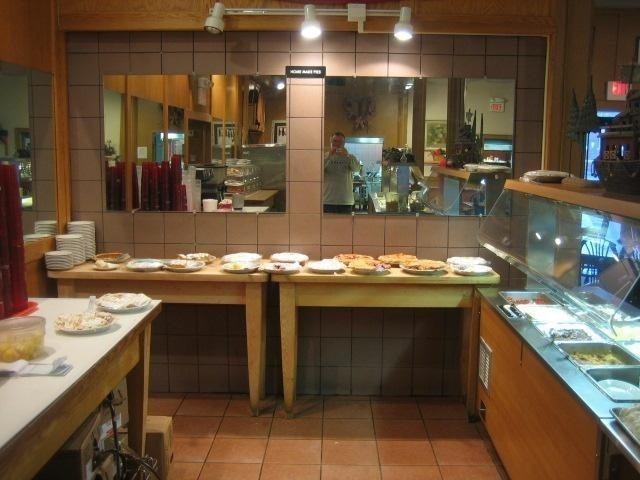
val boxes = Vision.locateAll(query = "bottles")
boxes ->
[406,148,415,162]
[399,148,407,162]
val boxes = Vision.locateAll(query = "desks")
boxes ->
[269,258,502,425]
[0,296,163,480]
[46,261,270,418]
[245,188,281,209]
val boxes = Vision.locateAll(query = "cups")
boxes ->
[202,199,218,212]
[231,190,244,211]
[218,202,232,212]
[141,152,202,212]
[385,192,400,212]
[105,159,139,209]
[245,176,262,194]
[1,163,29,319]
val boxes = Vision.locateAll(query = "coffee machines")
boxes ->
[199,164,228,203]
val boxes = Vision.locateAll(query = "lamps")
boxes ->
[203,2,415,43]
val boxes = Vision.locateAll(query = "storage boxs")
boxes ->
[143,413,175,480]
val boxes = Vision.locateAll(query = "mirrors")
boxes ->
[100,71,289,216]
[0,59,60,245]
[320,72,519,219]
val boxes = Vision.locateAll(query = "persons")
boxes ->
[324,132,360,213]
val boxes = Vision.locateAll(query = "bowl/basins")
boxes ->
[0,314,50,364]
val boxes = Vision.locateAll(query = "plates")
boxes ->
[306,261,344,273]
[398,261,446,274]
[52,312,115,336]
[96,264,118,271]
[95,290,150,312]
[462,164,509,174]
[163,260,205,273]
[43,220,95,270]
[450,266,493,276]
[523,172,563,184]
[93,252,128,264]
[261,263,301,274]
[23,220,53,245]
[482,159,507,167]
[222,252,262,262]
[597,378,640,397]
[348,260,393,274]
[126,258,162,273]
[448,255,489,265]
[270,251,308,263]
[176,253,216,263]
[221,264,260,273]
[225,158,252,165]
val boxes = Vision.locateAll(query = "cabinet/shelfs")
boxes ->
[472,179,640,479]
[417,161,512,221]
